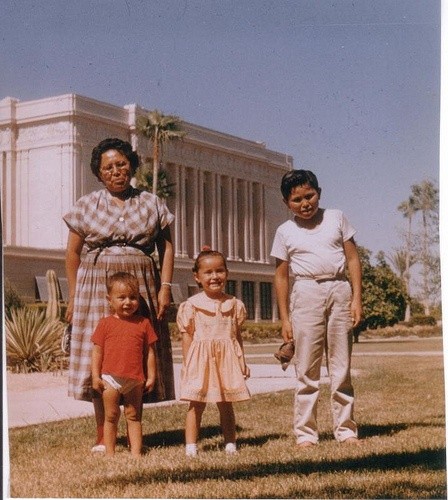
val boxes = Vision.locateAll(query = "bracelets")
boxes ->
[161,281,172,287]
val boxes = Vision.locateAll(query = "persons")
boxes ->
[176,250,251,458]
[63,138,179,456]
[90,273,158,457]
[270,169,363,449]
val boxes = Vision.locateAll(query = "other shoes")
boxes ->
[340,436,359,449]
[298,441,314,450]
[91,444,107,456]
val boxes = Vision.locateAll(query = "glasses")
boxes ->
[99,160,130,174]
